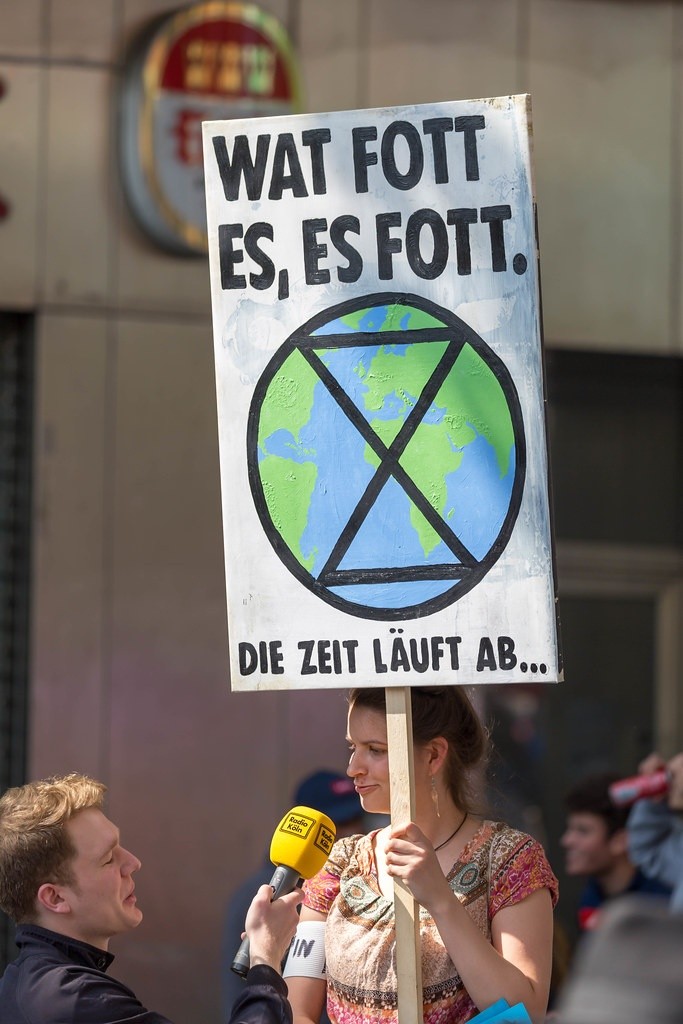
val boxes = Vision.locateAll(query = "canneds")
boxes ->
[609,768,669,808]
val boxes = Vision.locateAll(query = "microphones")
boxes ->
[231,805,338,976]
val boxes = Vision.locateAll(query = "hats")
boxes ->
[292,770,367,827]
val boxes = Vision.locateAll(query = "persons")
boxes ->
[275,686,561,1024]
[1,778,308,1024]
[219,770,367,1024]
[558,791,674,935]
[625,750,682,923]
